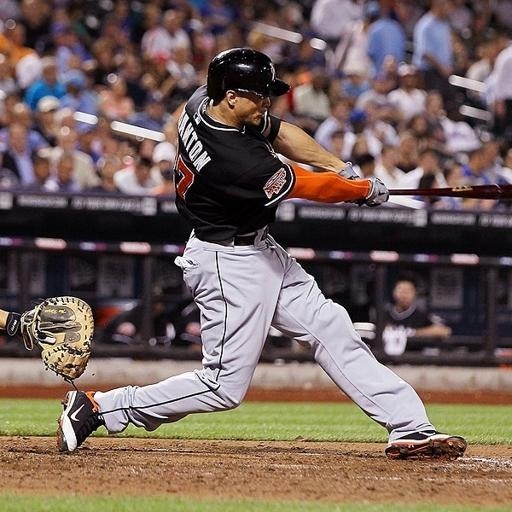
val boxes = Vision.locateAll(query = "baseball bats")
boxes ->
[388,184,512,200]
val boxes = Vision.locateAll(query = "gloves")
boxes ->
[336,162,360,180]
[364,176,389,207]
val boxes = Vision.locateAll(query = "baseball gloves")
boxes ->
[20,295,94,389]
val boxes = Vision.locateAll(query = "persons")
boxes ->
[97,280,452,359]
[57,48,467,461]
[0,1,512,208]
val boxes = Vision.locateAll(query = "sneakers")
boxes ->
[56,385,106,450]
[385,427,468,462]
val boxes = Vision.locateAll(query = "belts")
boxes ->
[235,225,271,246]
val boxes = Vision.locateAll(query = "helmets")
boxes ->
[205,47,292,101]
